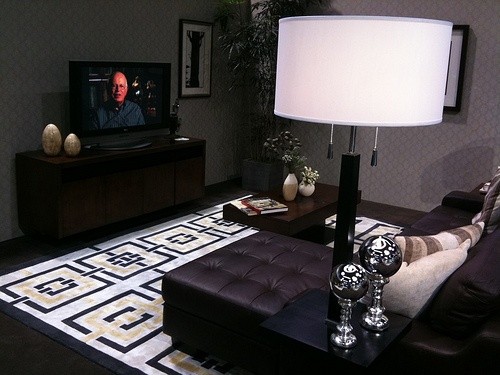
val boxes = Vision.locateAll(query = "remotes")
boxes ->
[243,208,258,217]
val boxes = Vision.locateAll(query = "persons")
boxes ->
[91,71,146,129]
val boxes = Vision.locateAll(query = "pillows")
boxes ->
[474,191,500,234]
[389,221,486,265]
[485,165,500,195]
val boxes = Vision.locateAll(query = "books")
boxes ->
[230,196,289,217]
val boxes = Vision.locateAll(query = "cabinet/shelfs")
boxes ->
[15,134,206,240]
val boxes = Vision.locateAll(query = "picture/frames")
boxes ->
[445,24,471,115]
[177,17,214,100]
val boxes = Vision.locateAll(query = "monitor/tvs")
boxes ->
[69,60,171,151]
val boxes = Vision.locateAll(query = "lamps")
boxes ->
[260,15,453,369]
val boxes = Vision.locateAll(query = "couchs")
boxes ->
[355,190,499,374]
[163,229,359,374]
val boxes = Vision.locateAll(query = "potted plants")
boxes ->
[206,0,333,193]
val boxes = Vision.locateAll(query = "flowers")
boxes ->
[263,131,307,174]
[300,166,319,186]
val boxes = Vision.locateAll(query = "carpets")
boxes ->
[0,194,409,375]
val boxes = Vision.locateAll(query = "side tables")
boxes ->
[259,290,412,375]
[469,182,488,197]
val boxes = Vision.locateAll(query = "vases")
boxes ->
[299,181,315,196]
[282,173,298,202]
